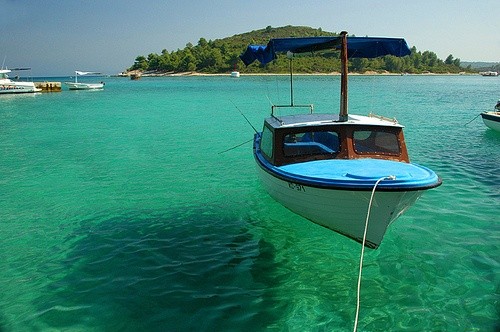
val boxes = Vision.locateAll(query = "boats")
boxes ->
[480,100,500,133]
[230,71,240,79]
[0,69,44,93]
[130,73,140,80]
[482,72,498,77]
[242,30,443,250]
[68,71,105,89]
